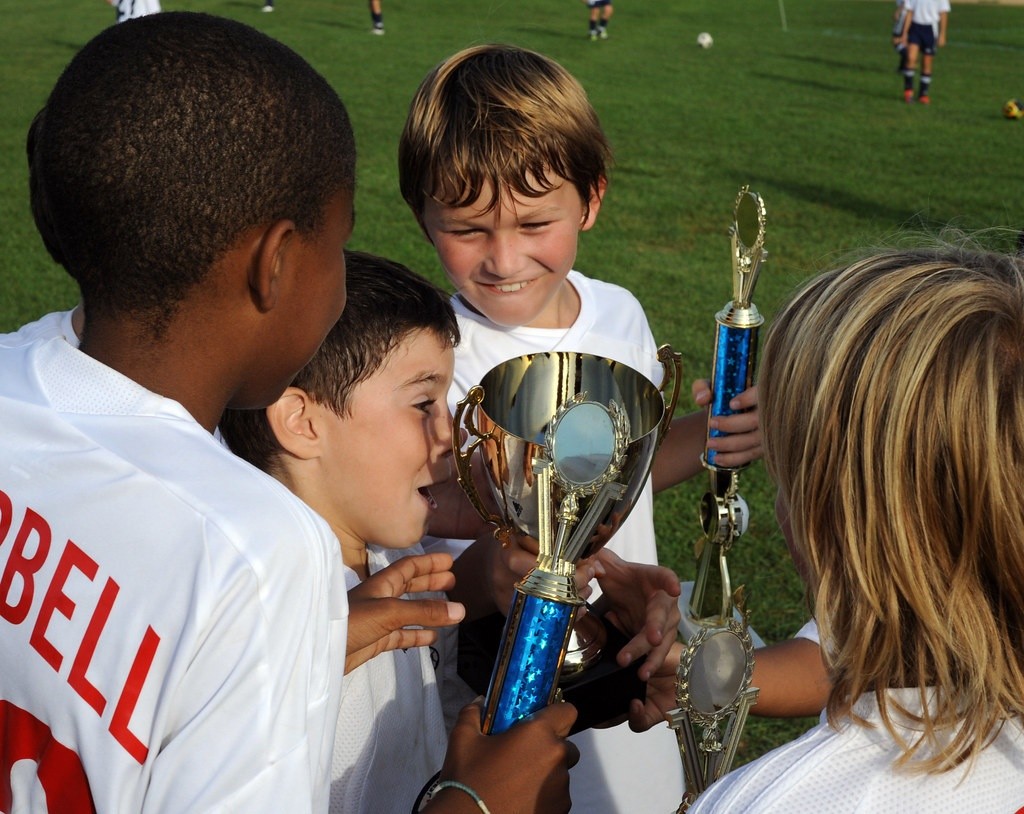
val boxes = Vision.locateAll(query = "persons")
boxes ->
[680,240,1024,812]
[608,617,848,736]
[366,43,768,813]
[366,0,386,36]
[261,0,276,13]
[582,0,615,42]
[890,1,916,75]
[896,0,952,105]
[108,0,163,24]
[207,251,683,812]
[0,11,586,812]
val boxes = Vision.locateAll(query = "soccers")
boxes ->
[1002,98,1024,121]
[697,32,713,49]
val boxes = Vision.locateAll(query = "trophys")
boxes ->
[662,616,760,814]
[663,174,777,664]
[448,343,685,746]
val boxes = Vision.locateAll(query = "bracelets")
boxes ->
[420,780,493,814]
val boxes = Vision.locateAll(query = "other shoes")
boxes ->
[919,96,930,104]
[261,4,272,12]
[587,31,598,42]
[371,28,384,35]
[598,26,610,40]
[902,90,916,101]
